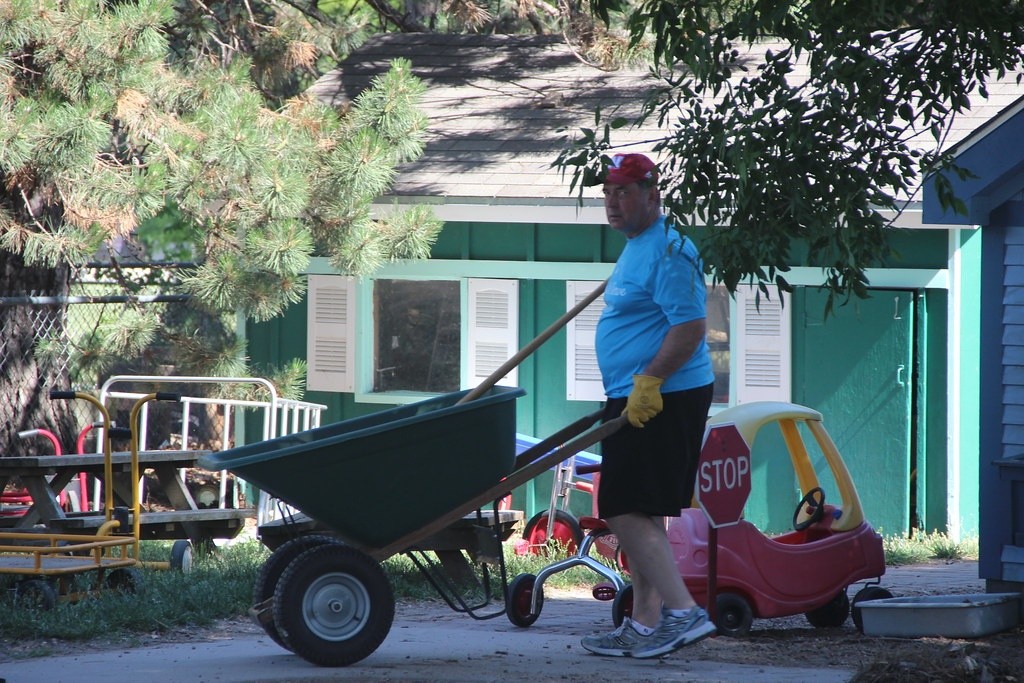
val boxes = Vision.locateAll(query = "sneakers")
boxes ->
[581,617,670,659]
[631,605,716,659]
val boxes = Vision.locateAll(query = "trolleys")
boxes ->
[1,389,197,611]
[198,380,632,667]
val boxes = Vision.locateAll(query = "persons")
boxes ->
[581,153,717,658]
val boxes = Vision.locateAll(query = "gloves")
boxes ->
[621,375,664,428]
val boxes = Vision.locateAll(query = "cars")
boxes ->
[669,401,895,640]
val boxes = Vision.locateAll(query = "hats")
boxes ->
[584,153,659,186]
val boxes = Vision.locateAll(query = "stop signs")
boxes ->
[694,420,754,530]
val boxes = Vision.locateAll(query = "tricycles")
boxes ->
[505,443,634,627]
